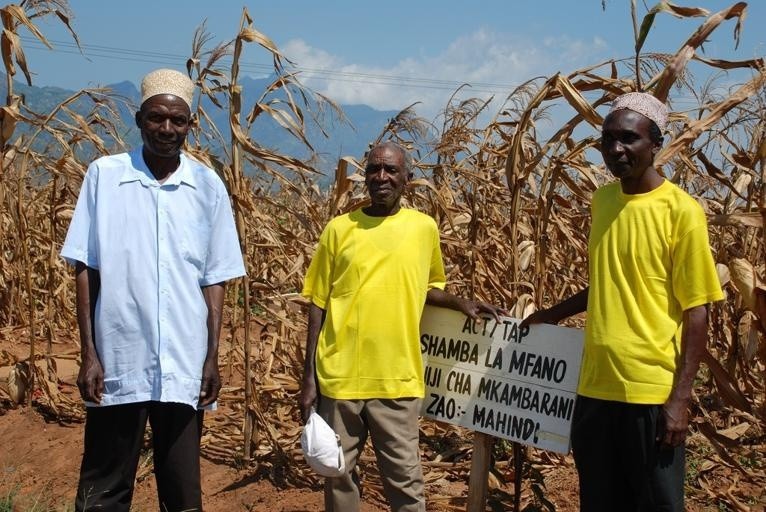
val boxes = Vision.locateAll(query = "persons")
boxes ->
[58,69,247,511]
[298,142,509,512]
[518,92,727,510]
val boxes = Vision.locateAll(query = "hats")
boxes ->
[602,92,670,137]
[298,403,346,479]
[140,68,195,113]
